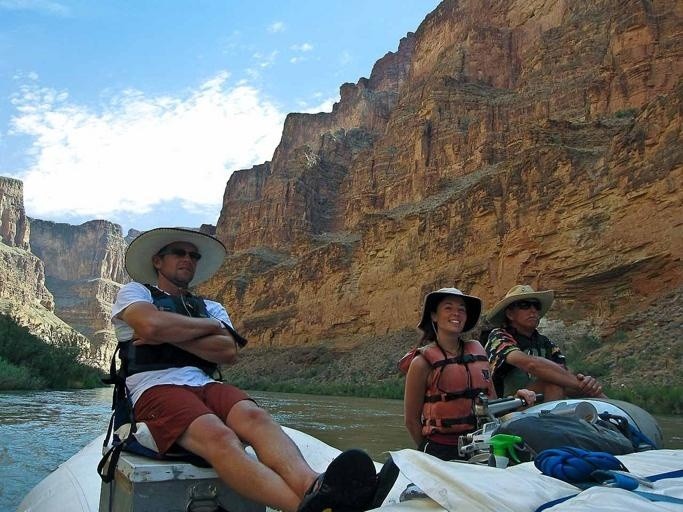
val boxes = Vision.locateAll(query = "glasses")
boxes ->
[158,248,202,262]
[511,299,542,312]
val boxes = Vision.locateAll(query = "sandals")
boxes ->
[297,449,400,512]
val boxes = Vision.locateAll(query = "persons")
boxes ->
[109,227,400,511]
[484,285,607,418]
[397,288,538,460]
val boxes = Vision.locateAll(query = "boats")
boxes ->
[12,412,445,511]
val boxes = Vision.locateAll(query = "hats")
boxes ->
[487,284,554,325]
[124,227,227,289]
[417,287,481,333]
